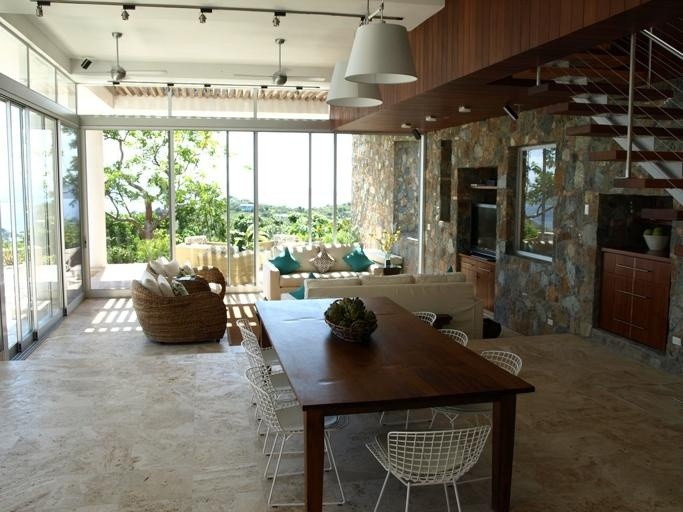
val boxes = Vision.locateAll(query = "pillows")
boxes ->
[268,246,374,272]
[141,256,196,296]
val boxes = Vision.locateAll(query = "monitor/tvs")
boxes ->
[471,203,497,261]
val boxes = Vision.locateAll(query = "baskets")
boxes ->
[324,299,377,343]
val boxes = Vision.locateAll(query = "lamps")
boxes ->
[412,128,421,140]
[272,38,288,86]
[30,0,403,29]
[81,56,92,70]
[326,0,419,109]
[502,104,523,121]
[111,31,126,80]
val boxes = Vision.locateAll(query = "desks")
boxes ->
[253,296,535,512]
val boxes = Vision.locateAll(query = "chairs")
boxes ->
[132,279,227,345]
[64,246,82,281]
[185,235,227,247]
[363,312,523,512]
[236,317,349,507]
[145,261,226,300]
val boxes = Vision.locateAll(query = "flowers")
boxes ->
[365,224,402,252]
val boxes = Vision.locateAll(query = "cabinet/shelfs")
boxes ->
[459,257,496,320]
[597,244,671,351]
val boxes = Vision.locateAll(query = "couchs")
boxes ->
[174,242,256,286]
[262,243,384,300]
[280,282,485,338]
